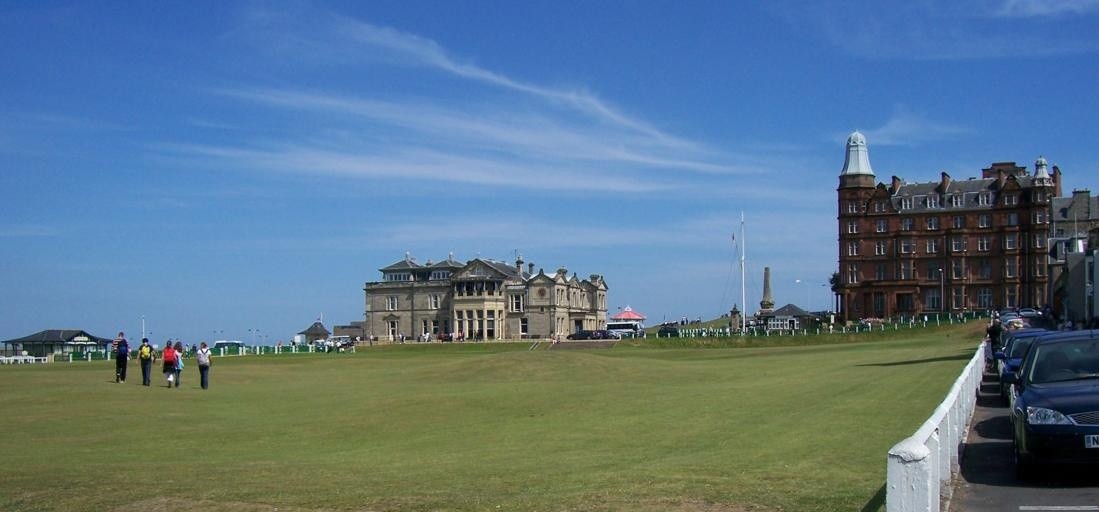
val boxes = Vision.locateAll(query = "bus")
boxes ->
[607,321,641,337]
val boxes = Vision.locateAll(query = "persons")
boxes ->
[424,327,483,343]
[987,318,1003,367]
[1044,306,1083,331]
[197,343,213,391]
[324,334,360,354]
[174,341,185,387]
[136,338,156,387]
[159,344,176,388]
[590,330,608,338]
[681,317,689,327]
[113,332,131,384]
[721,312,728,319]
[192,344,197,355]
[369,334,373,346]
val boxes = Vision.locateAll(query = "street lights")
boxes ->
[937,268,944,311]
[140,316,146,339]
[795,279,810,314]
[821,284,834,312]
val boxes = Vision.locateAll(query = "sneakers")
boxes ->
[116,373,120,383]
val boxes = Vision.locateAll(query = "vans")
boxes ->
[658,326,678,338]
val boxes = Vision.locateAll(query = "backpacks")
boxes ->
[198,349,210,366]
[140,345,151,360]
[117,339,128,356]
[175,353,184,370]
[164,349,175,362]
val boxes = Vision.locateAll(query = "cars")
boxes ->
[567,330,620,340]
[1001,328,1099,482]
[996,305,1064,402]
[861,316,885,327]
